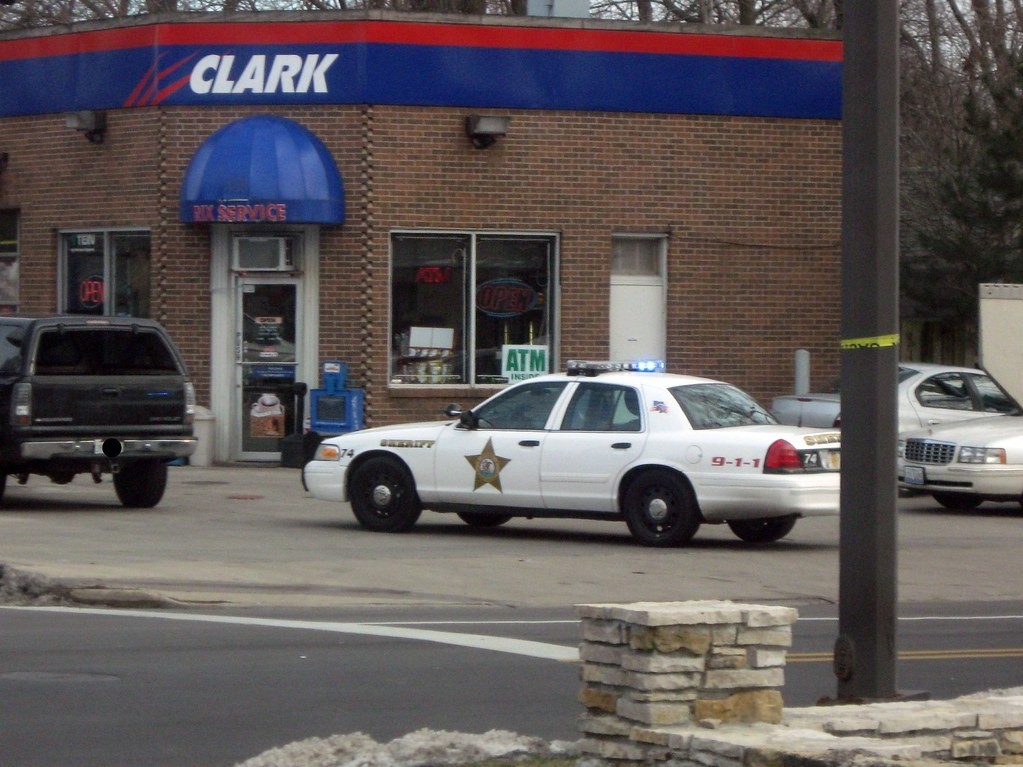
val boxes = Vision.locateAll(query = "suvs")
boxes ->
[0,312,200,508]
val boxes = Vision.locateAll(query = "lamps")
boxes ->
[65,110,107,143]
[463,114,512,148]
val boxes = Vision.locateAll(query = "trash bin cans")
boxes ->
[188,406,217,467]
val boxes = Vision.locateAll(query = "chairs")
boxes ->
[621,389,641,431]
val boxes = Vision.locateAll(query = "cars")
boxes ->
[298,359,842,551]
[896,413,1022,514]
[763,361,1015,495]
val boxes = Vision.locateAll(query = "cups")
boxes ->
[251,395,281,414]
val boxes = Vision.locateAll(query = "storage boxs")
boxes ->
[279,435,315,468]
